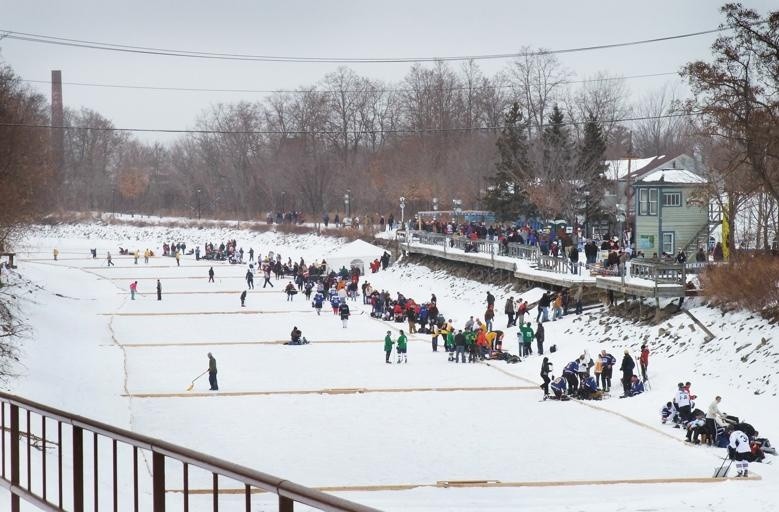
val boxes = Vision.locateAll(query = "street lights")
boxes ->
[112,187,116,214]
[432,198,439,222]
[280,191,286,212]
[452,199,462,225]
[399,197,406,222]
[197,188,202,220]
[615,202,626,285]
[344,188,354,218]
